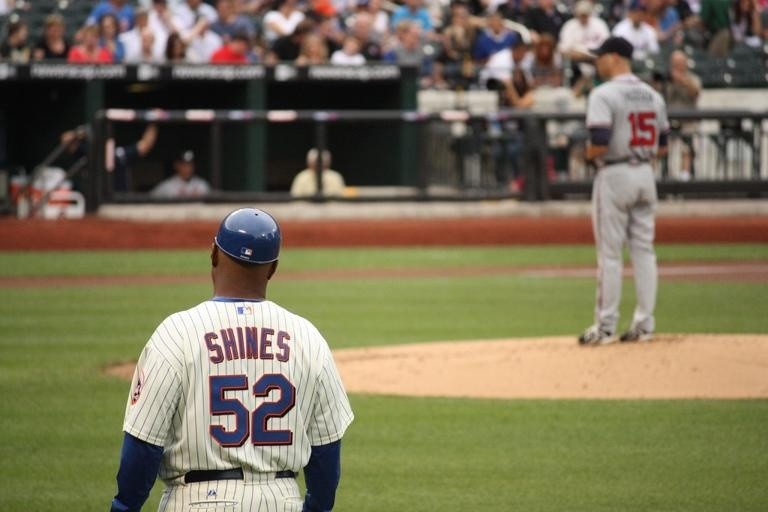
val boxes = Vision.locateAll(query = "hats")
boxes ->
[587,35,634,58]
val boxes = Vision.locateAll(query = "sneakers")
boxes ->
[619,327,655,342]
[578,327,618,346]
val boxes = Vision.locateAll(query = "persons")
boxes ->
[1,0,767,220]
[108,208,356,511]
[575,36,674,347]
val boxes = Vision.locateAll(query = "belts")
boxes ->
[181,466,296,484]
[606,155,650,166]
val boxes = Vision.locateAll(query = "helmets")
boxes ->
[215,205,283,265]
